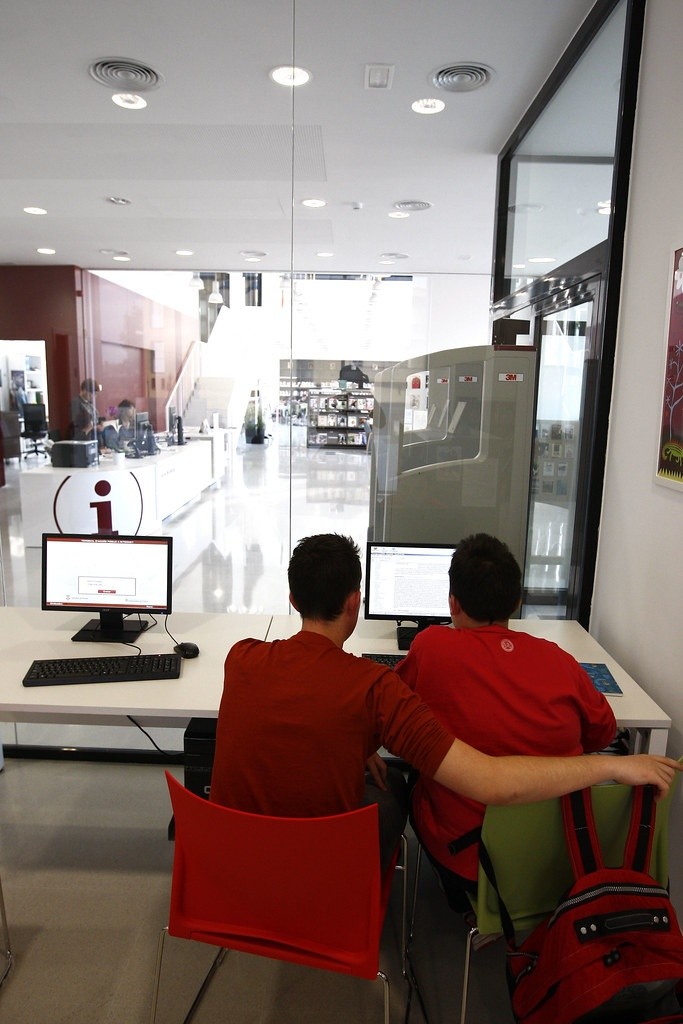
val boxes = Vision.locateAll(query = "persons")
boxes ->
[206,533,683,817]
[390,534,620,910]
[67,378,136,448]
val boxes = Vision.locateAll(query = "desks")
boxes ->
[0,604,672,765]
[19,426,228,549]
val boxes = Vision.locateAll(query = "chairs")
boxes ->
[148,769,419,1024]
[21,404,48,459]
[409,756,683,1024]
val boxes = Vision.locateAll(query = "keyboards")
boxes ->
[361,654,407,669]
[23,653,180,687]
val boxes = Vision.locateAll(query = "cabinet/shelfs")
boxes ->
[307,388,376,449]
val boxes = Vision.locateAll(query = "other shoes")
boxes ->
[463,910,504,951]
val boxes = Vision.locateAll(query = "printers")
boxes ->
[50,440,98,468]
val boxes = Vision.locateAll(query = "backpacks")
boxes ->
[506,783,683,1024]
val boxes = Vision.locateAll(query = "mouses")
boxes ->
[173,642,200,659]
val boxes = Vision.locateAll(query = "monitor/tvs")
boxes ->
[134,412,157,456]
[365,542,459,651]
[41,533,172,643]
[168,407,185,445]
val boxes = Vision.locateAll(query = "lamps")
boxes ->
[208,273,223,303]
[188,272,205,289]
